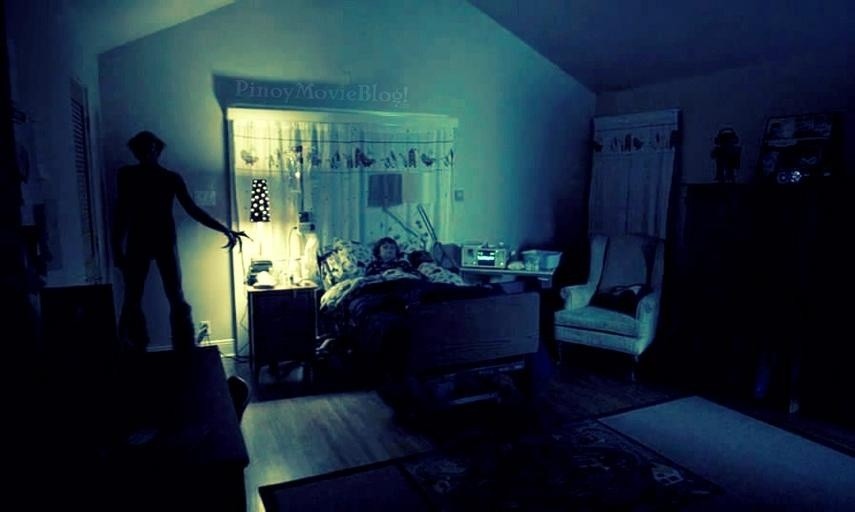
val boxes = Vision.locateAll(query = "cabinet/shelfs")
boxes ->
[1,343,251,510]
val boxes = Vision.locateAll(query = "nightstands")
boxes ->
[246,286,324,385]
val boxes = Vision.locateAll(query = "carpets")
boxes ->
[258,392,854,511]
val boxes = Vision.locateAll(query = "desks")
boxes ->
[459,266,557,284]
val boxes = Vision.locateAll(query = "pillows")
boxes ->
[320,237,425,285]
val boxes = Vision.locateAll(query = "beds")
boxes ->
[315,237,542,419]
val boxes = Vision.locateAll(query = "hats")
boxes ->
[126,131,166,153]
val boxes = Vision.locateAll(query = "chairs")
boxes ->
[551,229,668,384]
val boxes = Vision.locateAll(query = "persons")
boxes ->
[109,131,251,351]
[366,237,415,276]
